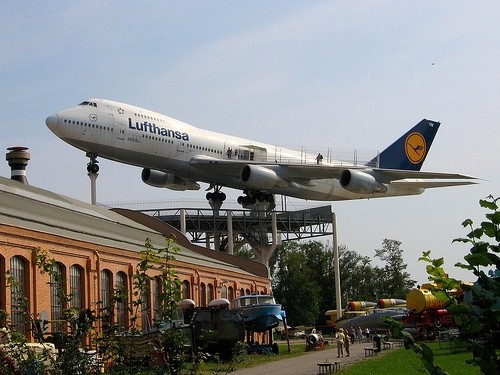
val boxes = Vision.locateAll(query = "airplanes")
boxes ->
[45,98,491,206]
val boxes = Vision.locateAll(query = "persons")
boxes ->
[336,328,345,358]
[343,327,372,348]
[344,330,352,357]
[226,146,324,166]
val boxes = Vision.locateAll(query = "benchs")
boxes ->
[364,347,378,357]
[383,342,393,349]
[317,360,341,375]
